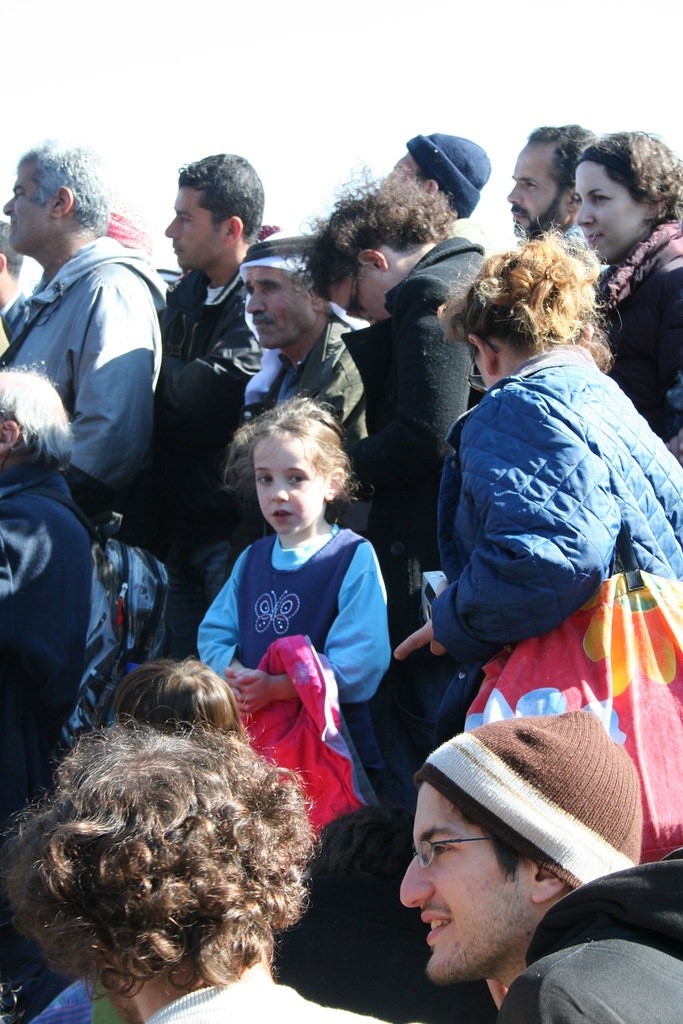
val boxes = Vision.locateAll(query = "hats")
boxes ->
[407,132,492,218]
[414,711,643,891]
[238,229,316,284]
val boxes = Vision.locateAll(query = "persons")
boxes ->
[400,709,683,1024]
[0,123,683,1024]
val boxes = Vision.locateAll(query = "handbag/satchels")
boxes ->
[483,564,681,863]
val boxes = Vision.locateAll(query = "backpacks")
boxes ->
[15,483,171,744]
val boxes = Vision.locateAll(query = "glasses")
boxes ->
[465,336,500,393]
[344,275,366,321]
[413,835,495,868]
